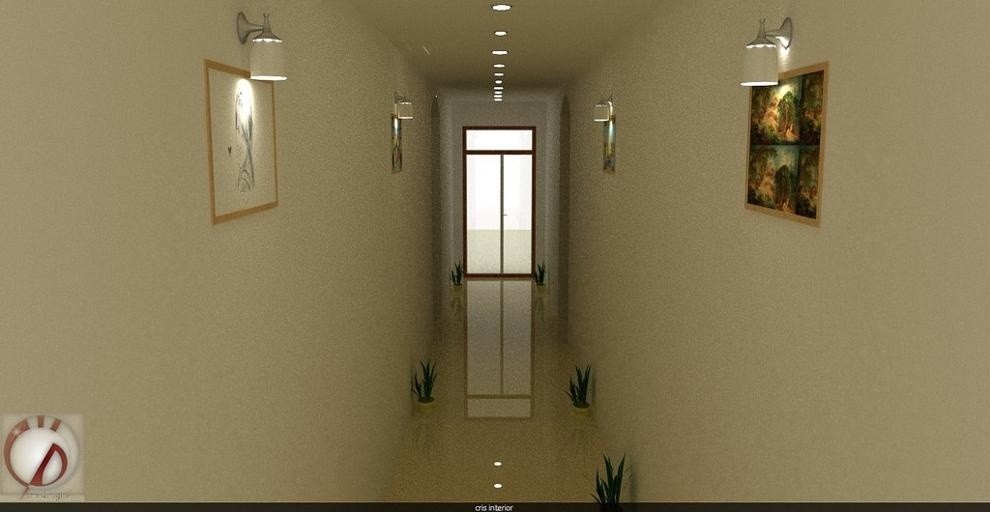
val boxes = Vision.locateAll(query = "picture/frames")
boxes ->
[742,59,830,232]
[203,58,281,225]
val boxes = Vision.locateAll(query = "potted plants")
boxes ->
[409,355,442,415]
[450,259,465,292]
[558,358,595,418]
[533,259,546,295]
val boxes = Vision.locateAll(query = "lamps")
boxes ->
[394,89,415,120]
[233,8,292,85]
[738,12,796,89]
[592,93,616,124]
